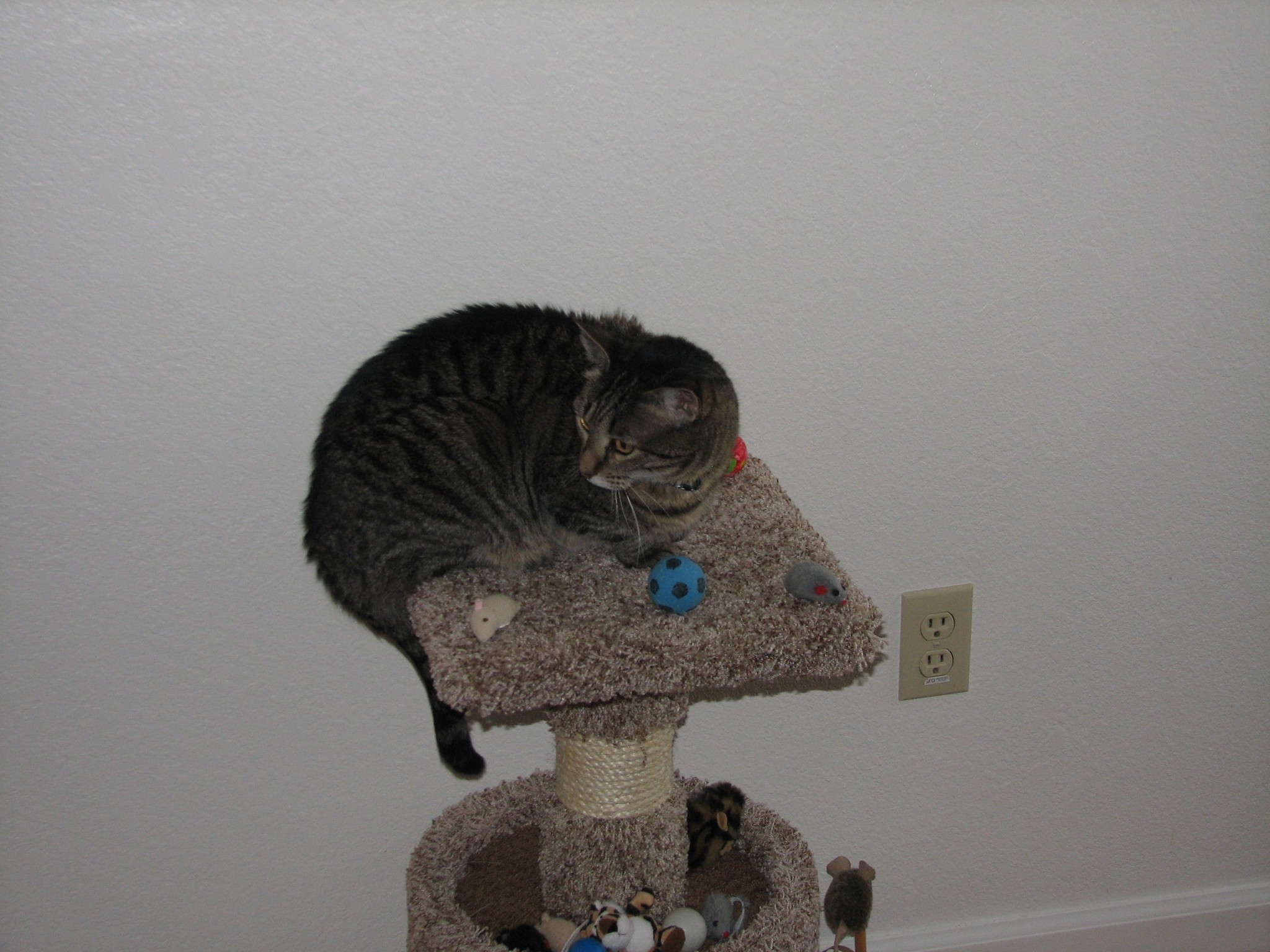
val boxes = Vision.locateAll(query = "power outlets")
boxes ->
[900,583,974,700]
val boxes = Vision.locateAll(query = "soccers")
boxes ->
[647,555,706,611]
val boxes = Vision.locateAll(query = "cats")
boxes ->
[303,302,740,782]
